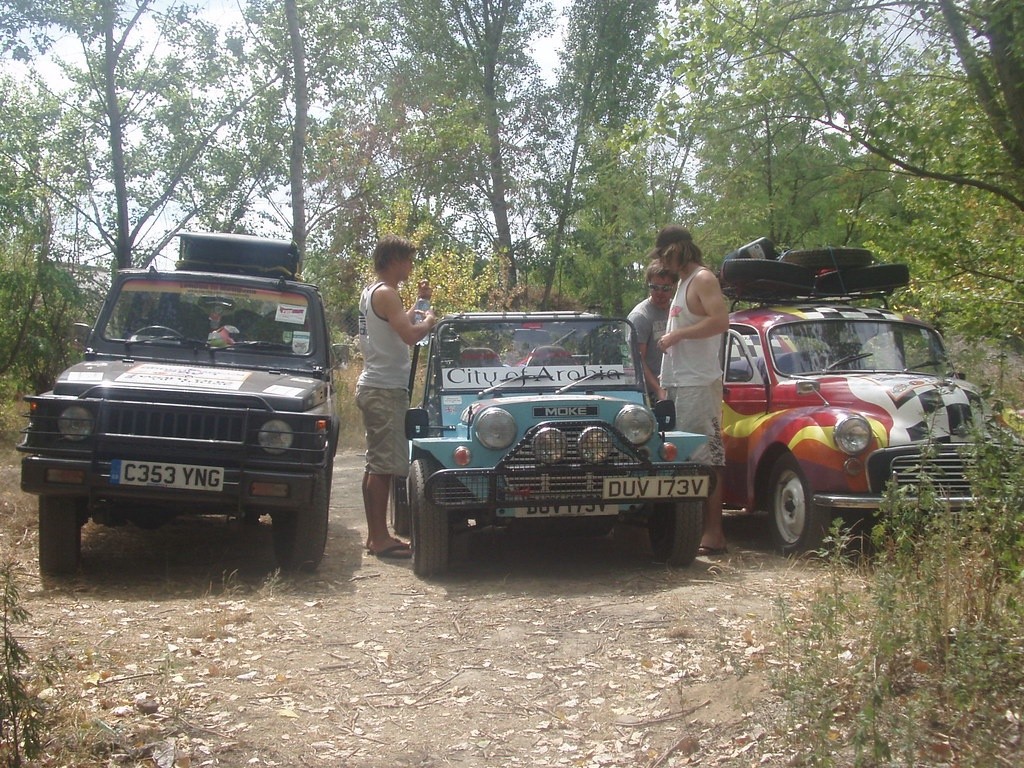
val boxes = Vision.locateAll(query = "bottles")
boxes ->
[414,297,431,347]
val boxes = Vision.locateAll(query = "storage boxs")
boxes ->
[174,232,299,281]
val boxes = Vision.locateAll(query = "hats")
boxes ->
[648,224,693,259]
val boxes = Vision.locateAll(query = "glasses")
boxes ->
[647,281,675,292]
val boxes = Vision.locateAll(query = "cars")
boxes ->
[716,305,1024,556]
[15,266,353,575]
[389,310,713,578]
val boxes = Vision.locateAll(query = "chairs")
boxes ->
[458,348,503,368]
[526,345,576,366]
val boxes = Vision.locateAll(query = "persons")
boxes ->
[355,236,437,554]
[624,257,677,408]
[646,225,729,556]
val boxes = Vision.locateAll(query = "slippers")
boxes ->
[697,546,727,557]
[367,538,412,559]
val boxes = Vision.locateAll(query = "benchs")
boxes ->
[776,350,851,374]
